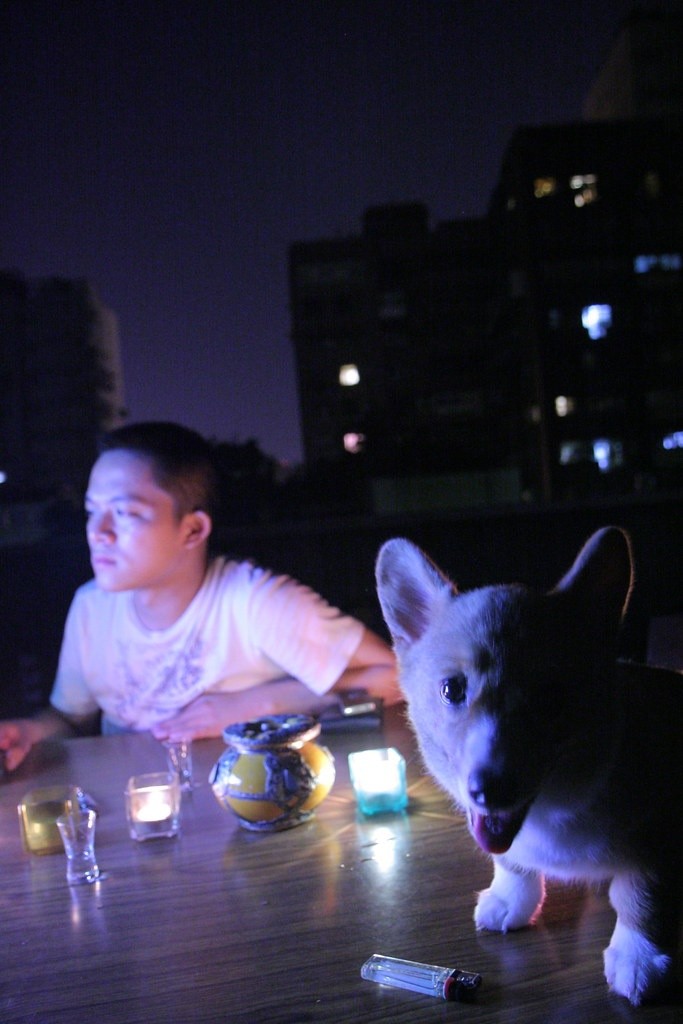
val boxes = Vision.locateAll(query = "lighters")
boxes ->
[358,952,484,1003]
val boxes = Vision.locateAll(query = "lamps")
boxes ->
[136,806,171,829]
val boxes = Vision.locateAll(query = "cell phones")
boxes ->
[337,688,377,717]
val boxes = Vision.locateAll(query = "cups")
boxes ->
[17,785,81,858]
[161,736,194,790]
[56,809,101,887]
[348,748,410,818]
[123,771,183,840]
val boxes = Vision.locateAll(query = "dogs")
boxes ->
[373,525,683,1008]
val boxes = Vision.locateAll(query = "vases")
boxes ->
[210,714,335,832]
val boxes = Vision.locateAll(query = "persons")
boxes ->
[0,420,405,773]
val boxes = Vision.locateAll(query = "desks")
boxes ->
[0,701,683,1024]
